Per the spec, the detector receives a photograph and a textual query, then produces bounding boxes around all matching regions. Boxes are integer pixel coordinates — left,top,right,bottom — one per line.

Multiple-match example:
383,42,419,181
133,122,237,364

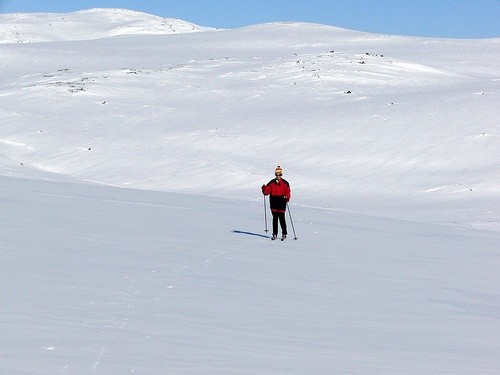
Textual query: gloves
285,197,289,203
262,185,266,191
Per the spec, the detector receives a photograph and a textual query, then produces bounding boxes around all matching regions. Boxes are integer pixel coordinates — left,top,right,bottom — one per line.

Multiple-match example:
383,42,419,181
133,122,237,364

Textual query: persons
261,165,291,241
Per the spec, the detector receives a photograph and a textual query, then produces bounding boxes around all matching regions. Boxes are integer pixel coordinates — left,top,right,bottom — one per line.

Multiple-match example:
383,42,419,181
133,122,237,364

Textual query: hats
275,166,283,173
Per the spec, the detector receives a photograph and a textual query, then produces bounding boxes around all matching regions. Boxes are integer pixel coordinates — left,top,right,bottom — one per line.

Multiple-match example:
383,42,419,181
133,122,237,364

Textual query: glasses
276,174,282,176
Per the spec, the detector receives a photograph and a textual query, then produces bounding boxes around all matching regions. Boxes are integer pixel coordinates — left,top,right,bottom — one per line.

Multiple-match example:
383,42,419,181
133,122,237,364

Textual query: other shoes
272,234,278,240
280,234,286,241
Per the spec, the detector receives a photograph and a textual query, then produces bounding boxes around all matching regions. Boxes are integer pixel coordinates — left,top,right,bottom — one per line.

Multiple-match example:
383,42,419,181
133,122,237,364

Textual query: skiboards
271,236,276,240
281,235,287,241
